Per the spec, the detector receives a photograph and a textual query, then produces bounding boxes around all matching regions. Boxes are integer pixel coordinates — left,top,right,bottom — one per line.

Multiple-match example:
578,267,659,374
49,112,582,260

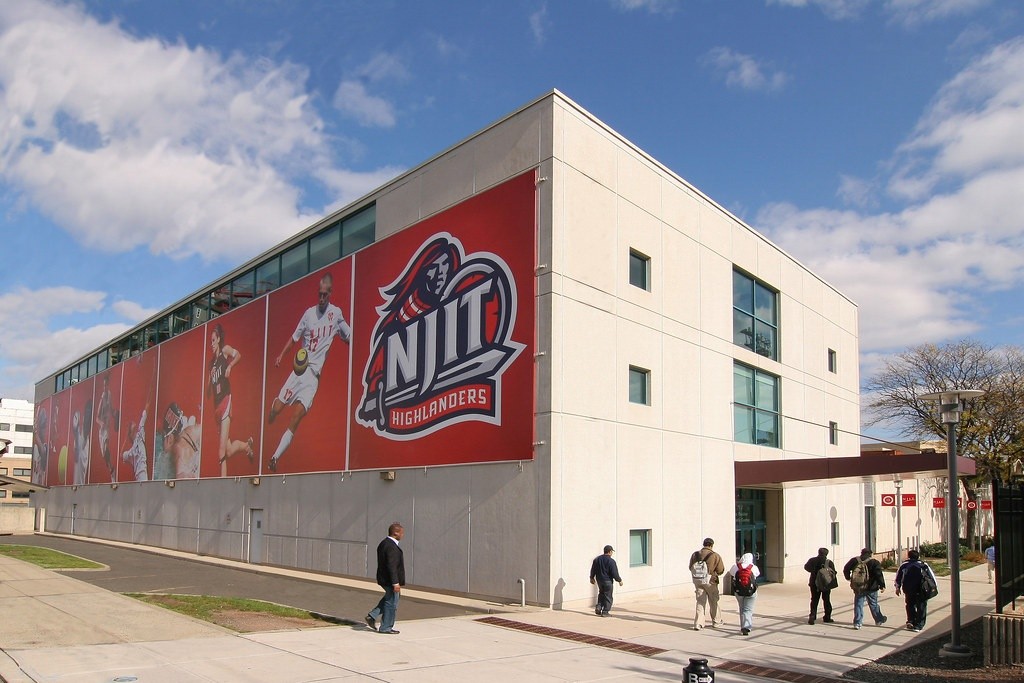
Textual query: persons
688,538,724,630
72,396,93,485
804,548,837,626
33,405,48,487
96,375,120,482
266,272,351,473
843,548,888,630
589,545,623,617
163,402,201,479
893,550,938,633
984,540,996,584
207,324,255,478
729,553,760,635
121,372,156,481
365,523,405,635
51,405,59,452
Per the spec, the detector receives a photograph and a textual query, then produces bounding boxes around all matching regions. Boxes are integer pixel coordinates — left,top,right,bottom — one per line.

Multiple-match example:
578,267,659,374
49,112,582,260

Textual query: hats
604,545,615,552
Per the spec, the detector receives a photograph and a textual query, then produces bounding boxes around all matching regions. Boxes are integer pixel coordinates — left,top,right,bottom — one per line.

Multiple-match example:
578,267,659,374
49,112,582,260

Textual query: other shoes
694,624,706,631
365,615,377,631
854,623,863,629
808,619,814,625
823,617,834,623
875,616,887,627
906,622,922,632
595,608,612,617
741,627,751,636
379,628,400,634
713,621,725,628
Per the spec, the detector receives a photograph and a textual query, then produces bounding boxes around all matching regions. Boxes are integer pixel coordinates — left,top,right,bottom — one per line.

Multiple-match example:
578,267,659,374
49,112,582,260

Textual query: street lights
971,488,987,554
894,479,904,567
917,389,986,658
942,481,948,566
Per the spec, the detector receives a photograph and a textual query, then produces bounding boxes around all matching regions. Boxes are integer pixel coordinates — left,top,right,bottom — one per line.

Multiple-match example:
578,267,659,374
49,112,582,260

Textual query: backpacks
732,564,758,596
850,556,874,591
815,559,838,592
691,551,714,585
911,563,938,601
